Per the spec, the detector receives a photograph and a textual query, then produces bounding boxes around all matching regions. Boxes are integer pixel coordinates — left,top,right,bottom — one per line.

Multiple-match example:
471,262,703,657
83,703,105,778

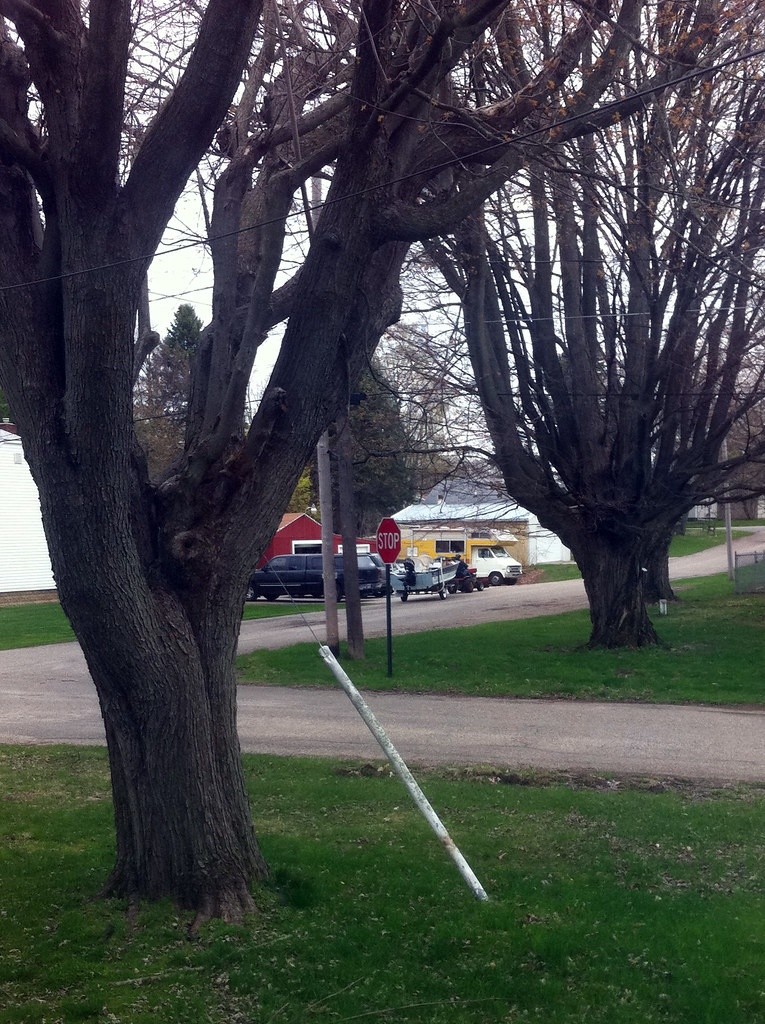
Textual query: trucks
398,527,522,586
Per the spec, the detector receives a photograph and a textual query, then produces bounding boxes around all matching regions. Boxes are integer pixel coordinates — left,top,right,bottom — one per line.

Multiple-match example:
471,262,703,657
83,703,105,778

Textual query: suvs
245,553,382,602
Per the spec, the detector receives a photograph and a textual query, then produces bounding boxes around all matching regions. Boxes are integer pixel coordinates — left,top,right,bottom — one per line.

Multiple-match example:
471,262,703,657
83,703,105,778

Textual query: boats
390,560,461,591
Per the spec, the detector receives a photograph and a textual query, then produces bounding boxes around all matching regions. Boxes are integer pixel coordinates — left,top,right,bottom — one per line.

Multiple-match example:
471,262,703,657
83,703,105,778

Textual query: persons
454,554,476,577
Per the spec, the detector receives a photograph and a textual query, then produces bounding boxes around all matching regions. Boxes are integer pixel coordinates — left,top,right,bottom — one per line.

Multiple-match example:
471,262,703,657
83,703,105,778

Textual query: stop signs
375,517,402,564
